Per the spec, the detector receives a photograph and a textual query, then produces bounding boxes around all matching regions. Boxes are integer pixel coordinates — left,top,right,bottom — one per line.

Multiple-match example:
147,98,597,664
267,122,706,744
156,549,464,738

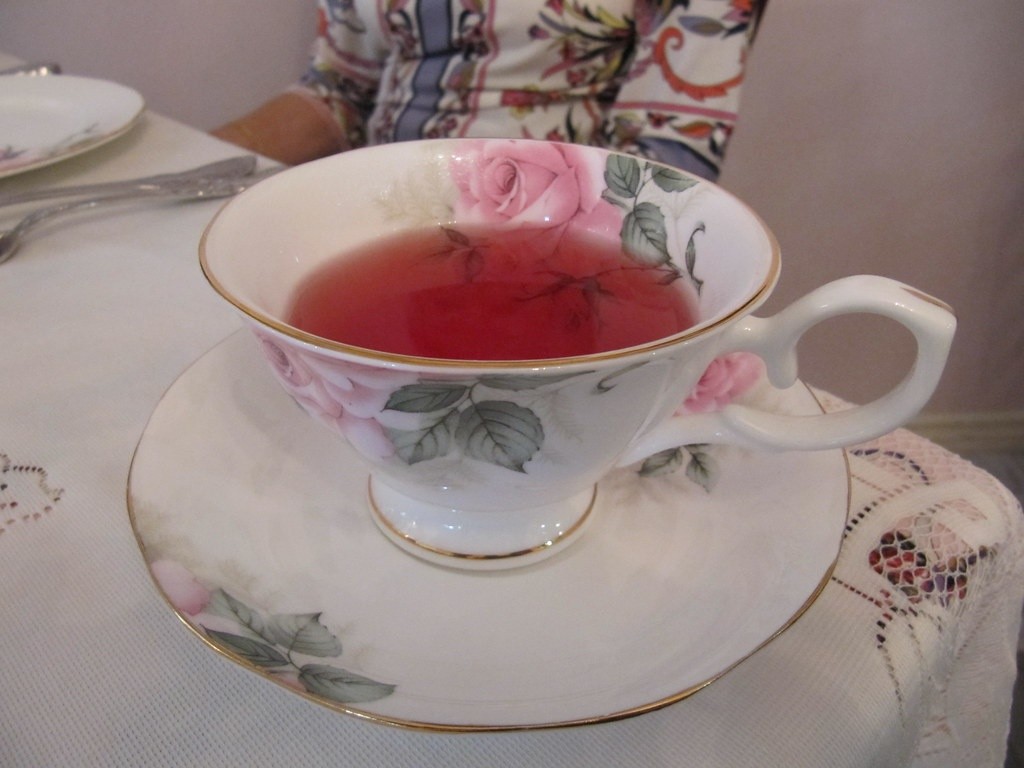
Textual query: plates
0,76,147,178
127,327,852,732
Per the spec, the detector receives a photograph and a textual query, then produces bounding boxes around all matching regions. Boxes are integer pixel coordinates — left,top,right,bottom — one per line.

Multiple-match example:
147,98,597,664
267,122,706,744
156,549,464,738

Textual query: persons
205,0,767,183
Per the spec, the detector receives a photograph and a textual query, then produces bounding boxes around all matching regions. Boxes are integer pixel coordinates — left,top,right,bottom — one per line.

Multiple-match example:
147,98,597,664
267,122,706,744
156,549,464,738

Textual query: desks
0,112,1024,768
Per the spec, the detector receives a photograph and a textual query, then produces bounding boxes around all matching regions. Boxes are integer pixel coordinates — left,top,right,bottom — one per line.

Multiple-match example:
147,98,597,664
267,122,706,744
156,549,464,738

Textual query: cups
198,137,958,570
